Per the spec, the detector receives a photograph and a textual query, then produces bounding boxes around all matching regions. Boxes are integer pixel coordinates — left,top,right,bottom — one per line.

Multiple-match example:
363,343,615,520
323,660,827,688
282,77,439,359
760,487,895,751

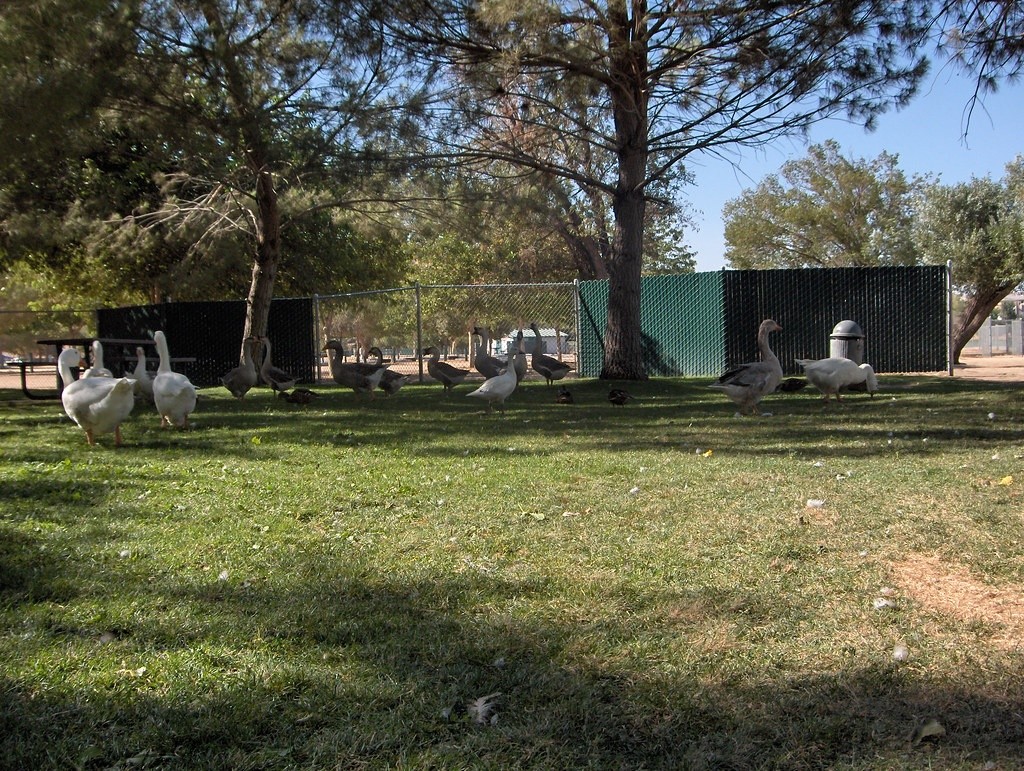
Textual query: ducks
56,317,877,448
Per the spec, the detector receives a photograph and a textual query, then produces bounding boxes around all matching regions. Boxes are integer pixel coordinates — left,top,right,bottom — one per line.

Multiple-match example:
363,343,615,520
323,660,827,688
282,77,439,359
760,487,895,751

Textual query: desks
37,337,157,401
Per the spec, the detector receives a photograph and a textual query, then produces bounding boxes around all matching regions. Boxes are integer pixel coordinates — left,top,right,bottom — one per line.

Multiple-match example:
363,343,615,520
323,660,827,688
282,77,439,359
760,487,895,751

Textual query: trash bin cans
830,319,865,366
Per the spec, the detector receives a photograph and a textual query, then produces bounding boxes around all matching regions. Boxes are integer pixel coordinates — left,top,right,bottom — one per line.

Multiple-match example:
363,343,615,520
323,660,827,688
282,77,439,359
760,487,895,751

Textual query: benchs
8,357,197,398
